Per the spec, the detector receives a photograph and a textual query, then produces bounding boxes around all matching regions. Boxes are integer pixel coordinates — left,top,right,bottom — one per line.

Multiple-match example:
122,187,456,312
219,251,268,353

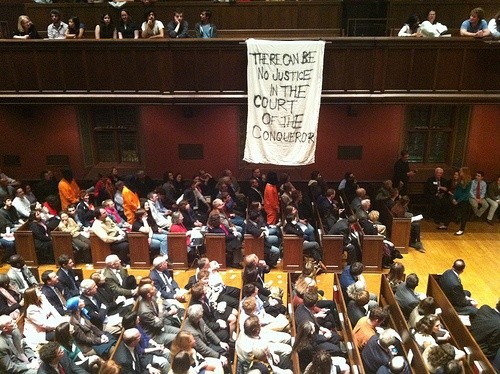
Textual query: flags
241,36,327,167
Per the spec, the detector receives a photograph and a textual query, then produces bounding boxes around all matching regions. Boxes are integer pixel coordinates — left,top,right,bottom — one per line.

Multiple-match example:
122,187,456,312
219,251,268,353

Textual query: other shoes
320,262,327,271
438,224,447,229
232,263,244,269
410,241,425,252
5,259,10,264
456,230,464,235
485,218,492,225
469,212,475,221
477,217,481,222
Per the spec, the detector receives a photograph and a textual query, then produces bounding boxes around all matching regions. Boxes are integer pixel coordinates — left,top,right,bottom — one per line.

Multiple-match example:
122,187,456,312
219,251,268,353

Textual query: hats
209,261,220,270
153,254,168,268
172,353,191,374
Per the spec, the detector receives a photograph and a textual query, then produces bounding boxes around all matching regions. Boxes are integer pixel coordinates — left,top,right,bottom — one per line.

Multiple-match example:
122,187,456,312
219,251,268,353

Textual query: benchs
15,192,500,373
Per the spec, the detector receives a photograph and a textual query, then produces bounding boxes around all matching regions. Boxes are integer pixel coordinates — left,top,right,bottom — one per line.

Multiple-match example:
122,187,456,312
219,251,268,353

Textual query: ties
21,268,33,288
92,296,100,309
162,272,177,294
58,363,66,374
54,286,67,307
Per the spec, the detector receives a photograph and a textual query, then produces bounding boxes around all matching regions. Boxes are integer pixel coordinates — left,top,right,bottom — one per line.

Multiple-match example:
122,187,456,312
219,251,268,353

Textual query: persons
451,166,472,236
425,168,454,229
420,8,448,38
485,176,500,226
469,171,489,221
398,14,424,38
447,171,463,222
460,7,491,38
488,11,500,37
0,165,500,374
11,0,219,41
391,149,418,196
